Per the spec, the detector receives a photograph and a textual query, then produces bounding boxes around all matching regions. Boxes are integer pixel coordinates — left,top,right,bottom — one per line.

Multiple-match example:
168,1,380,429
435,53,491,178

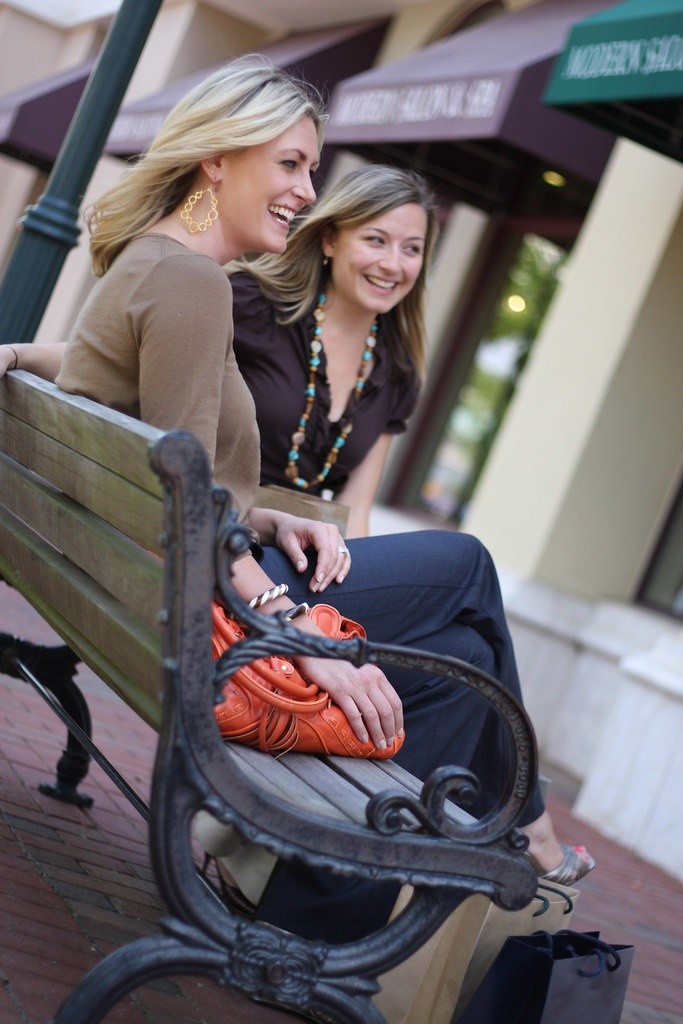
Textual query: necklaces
286,286,378,493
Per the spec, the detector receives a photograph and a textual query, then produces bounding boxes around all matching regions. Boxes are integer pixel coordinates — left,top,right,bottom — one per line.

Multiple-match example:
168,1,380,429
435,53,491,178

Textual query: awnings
0,56,96,170
309,0,610,210
540,0,683,159
104,18,386,157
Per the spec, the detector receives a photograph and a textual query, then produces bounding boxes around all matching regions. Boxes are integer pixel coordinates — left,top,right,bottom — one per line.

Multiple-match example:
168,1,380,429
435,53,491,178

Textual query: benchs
0,363,540,1024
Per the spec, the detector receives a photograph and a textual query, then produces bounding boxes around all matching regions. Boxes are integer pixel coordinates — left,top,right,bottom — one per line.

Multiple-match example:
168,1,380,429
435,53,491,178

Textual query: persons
0,59,598,1004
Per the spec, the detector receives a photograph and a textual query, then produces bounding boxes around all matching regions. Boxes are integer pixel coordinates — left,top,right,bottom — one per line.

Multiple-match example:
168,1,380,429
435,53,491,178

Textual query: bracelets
246,584,310,624
4,344,20,370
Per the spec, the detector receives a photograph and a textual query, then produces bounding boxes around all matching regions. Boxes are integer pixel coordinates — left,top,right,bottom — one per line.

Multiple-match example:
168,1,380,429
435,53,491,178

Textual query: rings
339,549,348,555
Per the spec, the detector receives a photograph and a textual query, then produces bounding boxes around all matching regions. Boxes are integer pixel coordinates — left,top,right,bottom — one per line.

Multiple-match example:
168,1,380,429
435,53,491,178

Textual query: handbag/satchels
210,598,406,760
456,928,636,1024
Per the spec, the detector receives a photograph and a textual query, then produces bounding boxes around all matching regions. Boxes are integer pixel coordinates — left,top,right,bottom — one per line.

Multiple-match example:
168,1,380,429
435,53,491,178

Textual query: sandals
537,845,597,888
